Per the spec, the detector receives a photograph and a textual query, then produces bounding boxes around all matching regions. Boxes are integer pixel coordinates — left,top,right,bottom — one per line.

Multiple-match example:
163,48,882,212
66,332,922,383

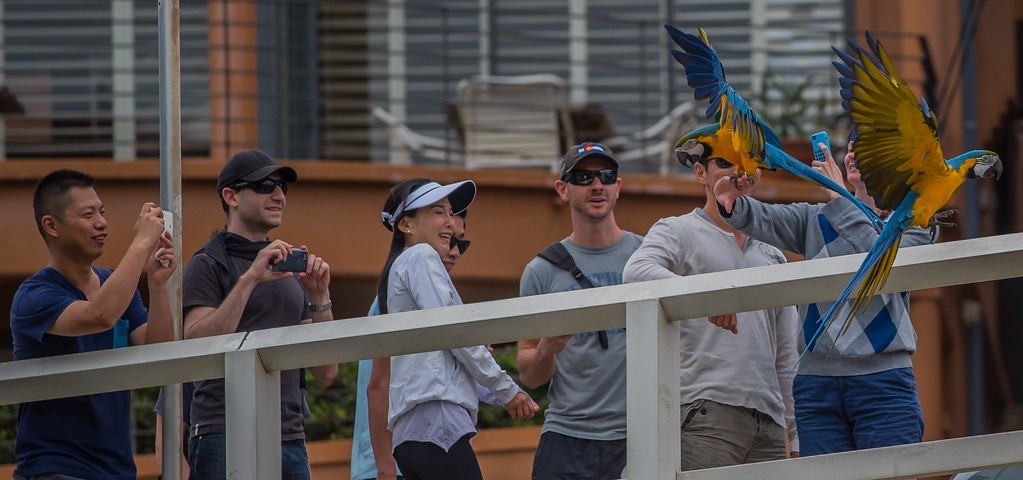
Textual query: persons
182,149,340,480
510,141,648,480
351,205,471,480
9,167,177,480
622,138,803,473
710,123,941,459
382,177,541,480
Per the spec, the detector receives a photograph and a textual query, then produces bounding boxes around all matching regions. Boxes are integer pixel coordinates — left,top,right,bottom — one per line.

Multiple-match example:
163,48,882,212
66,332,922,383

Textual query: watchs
308,298,332,314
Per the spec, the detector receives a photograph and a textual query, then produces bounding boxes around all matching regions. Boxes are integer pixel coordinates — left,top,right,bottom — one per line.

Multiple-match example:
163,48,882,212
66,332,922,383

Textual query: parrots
786,31,1003,379
664,24,886,236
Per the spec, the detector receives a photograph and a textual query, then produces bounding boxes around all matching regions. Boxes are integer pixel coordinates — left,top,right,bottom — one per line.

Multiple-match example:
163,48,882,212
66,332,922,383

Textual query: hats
381,180,476,231
560,141,620,182
215,149,296,195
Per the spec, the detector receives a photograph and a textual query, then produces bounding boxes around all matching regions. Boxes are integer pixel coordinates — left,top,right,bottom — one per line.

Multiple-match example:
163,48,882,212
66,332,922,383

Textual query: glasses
711,158,733,170
230,179,288,195
449,235,471,256
562,168,618,186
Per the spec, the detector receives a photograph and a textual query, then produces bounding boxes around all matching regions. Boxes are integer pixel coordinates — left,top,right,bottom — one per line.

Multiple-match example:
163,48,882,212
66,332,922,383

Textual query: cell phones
277,248,308,272
149,207,173,239
811,131,831,162
